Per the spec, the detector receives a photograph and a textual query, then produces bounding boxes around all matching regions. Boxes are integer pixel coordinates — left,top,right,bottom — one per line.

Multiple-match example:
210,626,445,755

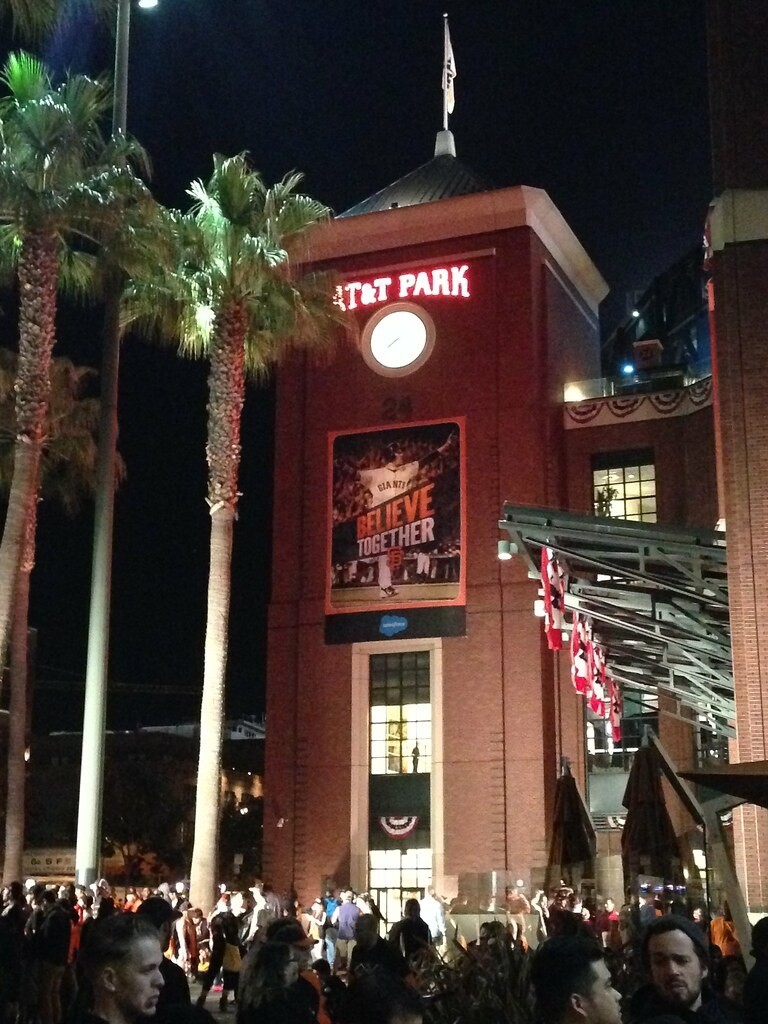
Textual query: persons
333,430,460,598
1,879,768,1024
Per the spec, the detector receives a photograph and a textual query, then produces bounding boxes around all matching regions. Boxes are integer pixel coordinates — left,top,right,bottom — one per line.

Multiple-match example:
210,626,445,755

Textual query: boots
220,998,235,1013
197,997,206,1008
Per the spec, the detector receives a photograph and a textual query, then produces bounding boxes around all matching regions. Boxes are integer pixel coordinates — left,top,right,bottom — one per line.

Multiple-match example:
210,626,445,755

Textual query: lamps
498,540,518,560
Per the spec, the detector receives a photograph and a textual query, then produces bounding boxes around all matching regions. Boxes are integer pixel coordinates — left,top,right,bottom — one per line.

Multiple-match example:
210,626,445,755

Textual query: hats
266,917,319,947
137,897,182,928
312,959,330,976
641,914,711,963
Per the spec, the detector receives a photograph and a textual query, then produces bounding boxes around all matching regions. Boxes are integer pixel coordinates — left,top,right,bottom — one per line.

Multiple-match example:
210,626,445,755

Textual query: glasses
293,945,310,951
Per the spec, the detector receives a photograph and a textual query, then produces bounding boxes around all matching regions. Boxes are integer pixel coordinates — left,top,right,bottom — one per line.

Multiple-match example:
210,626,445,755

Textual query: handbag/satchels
223,943,241,972
